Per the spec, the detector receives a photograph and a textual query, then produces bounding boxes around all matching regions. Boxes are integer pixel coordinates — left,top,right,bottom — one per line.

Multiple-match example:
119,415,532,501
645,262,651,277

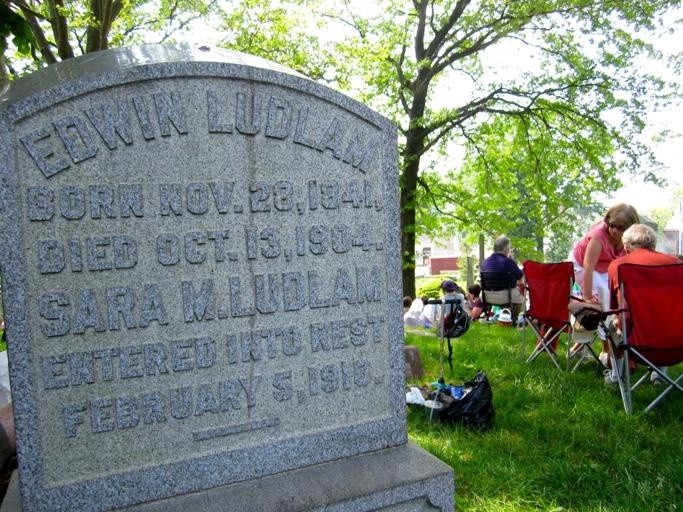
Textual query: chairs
481,261,683,414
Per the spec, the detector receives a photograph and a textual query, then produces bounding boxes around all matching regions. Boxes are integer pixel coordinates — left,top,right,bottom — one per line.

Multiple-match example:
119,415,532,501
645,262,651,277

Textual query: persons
601,222,682,387
400,278,484,322
566,203,640,368
479,235,526,326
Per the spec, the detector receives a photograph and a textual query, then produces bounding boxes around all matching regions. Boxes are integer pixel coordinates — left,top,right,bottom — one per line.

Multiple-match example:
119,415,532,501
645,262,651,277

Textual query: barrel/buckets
497,309,512,327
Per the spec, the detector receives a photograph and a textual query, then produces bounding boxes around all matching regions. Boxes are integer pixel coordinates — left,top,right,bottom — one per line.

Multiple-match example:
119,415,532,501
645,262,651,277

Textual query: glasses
607,222,625,230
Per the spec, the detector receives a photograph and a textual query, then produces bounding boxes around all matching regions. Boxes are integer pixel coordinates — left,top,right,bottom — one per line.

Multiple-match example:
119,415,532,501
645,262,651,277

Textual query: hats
441,280,458,292
570,314,594,343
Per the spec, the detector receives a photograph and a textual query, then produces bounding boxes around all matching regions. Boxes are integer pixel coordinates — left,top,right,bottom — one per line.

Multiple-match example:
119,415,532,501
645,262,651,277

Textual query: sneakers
598,352,607,368
602,369,621,386
650,370,668,386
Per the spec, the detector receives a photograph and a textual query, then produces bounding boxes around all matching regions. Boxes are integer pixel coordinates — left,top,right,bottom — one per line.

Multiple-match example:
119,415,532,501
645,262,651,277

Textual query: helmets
436,299,471,338
